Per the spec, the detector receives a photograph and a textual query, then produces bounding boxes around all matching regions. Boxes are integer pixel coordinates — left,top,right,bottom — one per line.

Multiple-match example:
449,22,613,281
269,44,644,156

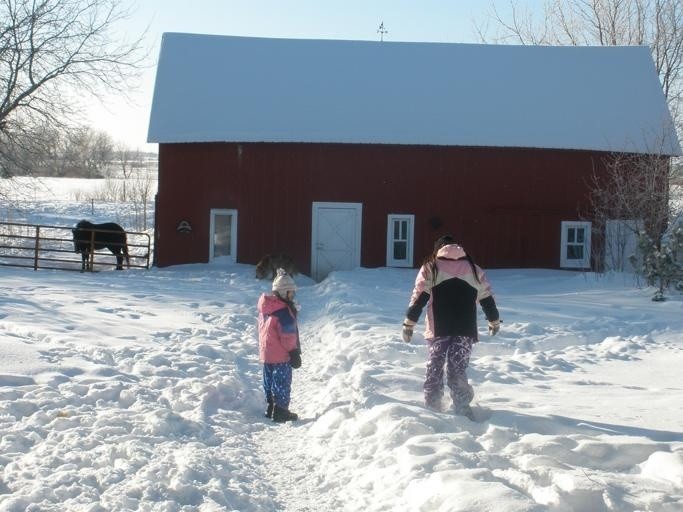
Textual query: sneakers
264,403,298,422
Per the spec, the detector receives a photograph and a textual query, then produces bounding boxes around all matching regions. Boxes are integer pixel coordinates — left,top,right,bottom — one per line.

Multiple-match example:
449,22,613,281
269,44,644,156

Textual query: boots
423,389,475,423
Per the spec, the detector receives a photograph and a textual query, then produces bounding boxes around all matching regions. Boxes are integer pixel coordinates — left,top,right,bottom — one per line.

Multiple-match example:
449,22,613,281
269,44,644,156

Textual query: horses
71,220,131,271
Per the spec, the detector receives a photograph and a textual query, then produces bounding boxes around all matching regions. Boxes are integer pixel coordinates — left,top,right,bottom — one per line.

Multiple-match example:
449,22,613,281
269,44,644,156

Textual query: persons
397,233,502,415
254,270,302,423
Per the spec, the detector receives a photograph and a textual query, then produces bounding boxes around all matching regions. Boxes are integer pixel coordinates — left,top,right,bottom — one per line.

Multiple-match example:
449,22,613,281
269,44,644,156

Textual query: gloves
289,348,302,369
402,317,418,343
487,318,501,337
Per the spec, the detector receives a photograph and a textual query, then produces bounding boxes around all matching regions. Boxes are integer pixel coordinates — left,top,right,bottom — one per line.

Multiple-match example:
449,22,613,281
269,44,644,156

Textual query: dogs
255,253,300,282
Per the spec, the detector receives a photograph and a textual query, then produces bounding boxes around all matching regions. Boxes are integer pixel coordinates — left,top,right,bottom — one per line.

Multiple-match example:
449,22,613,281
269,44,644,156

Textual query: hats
272,268,298,303
